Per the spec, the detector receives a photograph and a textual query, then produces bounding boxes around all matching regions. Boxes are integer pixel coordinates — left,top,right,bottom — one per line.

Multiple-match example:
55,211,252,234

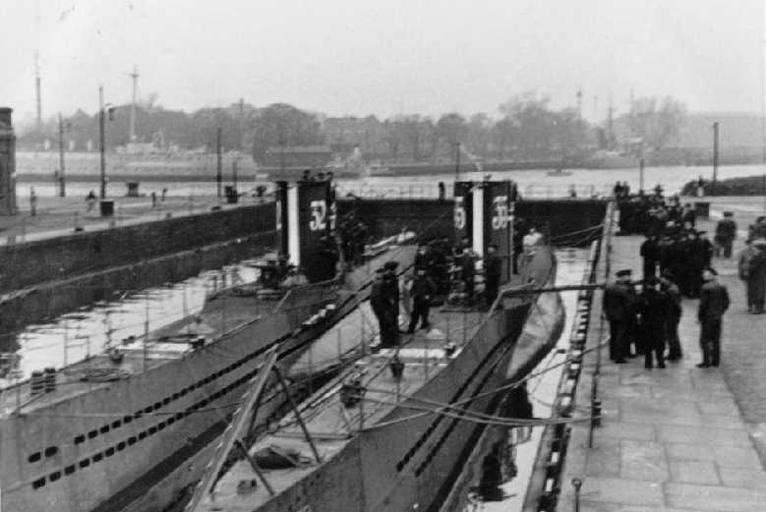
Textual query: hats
706,266,717,276
417,266,426,270
374,262,398,280
615,269,673,286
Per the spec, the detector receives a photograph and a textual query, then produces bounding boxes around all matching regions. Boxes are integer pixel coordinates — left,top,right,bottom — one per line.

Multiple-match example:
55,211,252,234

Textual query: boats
180,179,560,511
0,174,431,511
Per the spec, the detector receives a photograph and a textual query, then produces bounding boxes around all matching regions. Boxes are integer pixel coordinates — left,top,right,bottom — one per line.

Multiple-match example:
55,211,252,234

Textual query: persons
602,175,766,374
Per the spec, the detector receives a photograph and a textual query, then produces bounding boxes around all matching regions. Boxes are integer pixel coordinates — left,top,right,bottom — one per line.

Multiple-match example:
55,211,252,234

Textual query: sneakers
406,322,428,334
610,352,681,368
699,360,718,367
